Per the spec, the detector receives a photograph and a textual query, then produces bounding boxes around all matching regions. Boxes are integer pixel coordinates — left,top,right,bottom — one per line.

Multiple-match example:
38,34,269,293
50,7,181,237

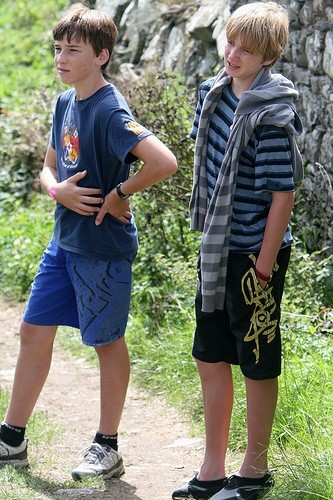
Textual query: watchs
255,268,272,282
116,182,133,200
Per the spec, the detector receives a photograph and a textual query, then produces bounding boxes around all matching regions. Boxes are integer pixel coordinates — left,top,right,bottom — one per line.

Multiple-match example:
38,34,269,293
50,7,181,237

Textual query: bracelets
50,184,56,201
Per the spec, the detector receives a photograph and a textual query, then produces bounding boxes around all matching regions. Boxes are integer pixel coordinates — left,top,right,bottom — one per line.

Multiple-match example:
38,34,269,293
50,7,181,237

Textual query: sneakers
72,441,125,480
0,438,29,466
172,477,229,500
209,473,275,500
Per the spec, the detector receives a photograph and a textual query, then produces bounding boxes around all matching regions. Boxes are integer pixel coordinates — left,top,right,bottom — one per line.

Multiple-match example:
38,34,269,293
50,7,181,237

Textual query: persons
0,4,178,482
171,2,304,500
